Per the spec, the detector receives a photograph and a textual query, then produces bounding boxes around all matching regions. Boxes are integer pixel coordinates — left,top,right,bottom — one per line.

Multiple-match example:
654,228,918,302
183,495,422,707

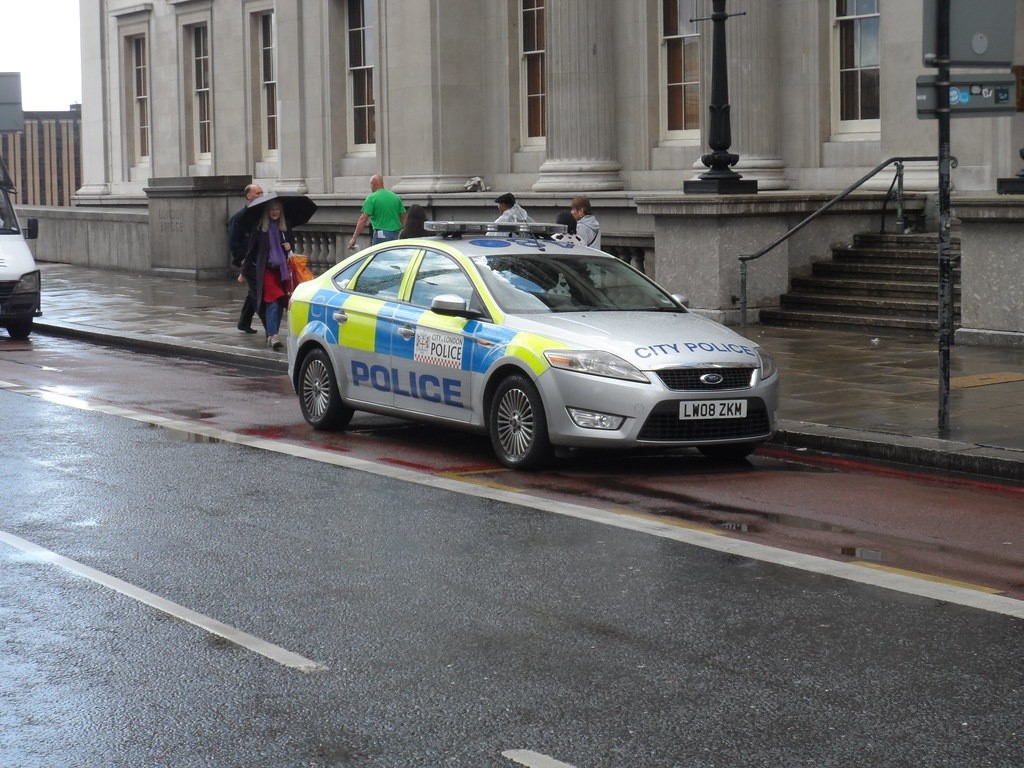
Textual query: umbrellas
244,191,319,243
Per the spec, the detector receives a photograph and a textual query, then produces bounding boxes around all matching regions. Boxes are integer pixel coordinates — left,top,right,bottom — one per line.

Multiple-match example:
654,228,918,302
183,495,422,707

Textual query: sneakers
268,334,283,348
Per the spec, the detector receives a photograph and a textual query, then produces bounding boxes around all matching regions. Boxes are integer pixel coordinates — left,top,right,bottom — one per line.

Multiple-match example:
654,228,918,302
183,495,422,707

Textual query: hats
495,193,515,206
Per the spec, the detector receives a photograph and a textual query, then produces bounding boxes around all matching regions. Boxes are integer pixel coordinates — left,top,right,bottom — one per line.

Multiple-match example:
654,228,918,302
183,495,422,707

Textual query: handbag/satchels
286,250,314,298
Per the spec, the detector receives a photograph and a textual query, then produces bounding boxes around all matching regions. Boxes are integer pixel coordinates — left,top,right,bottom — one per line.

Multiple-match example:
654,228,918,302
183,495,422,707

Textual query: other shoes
238,325,257,334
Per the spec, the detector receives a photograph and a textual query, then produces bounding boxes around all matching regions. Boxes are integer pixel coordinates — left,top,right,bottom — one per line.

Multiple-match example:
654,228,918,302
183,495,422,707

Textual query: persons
398,204,436,240
348,174,407,250
486,193,529,277
549,197,601,296
229,185,267,333
238,199,295,348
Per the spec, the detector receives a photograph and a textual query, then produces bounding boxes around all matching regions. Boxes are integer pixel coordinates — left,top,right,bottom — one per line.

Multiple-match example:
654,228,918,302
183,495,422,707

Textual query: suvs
0,156,41,342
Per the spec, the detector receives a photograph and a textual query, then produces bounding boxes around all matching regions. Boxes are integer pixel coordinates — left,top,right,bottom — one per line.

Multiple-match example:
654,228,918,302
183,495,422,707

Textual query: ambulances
287,221,779,470
